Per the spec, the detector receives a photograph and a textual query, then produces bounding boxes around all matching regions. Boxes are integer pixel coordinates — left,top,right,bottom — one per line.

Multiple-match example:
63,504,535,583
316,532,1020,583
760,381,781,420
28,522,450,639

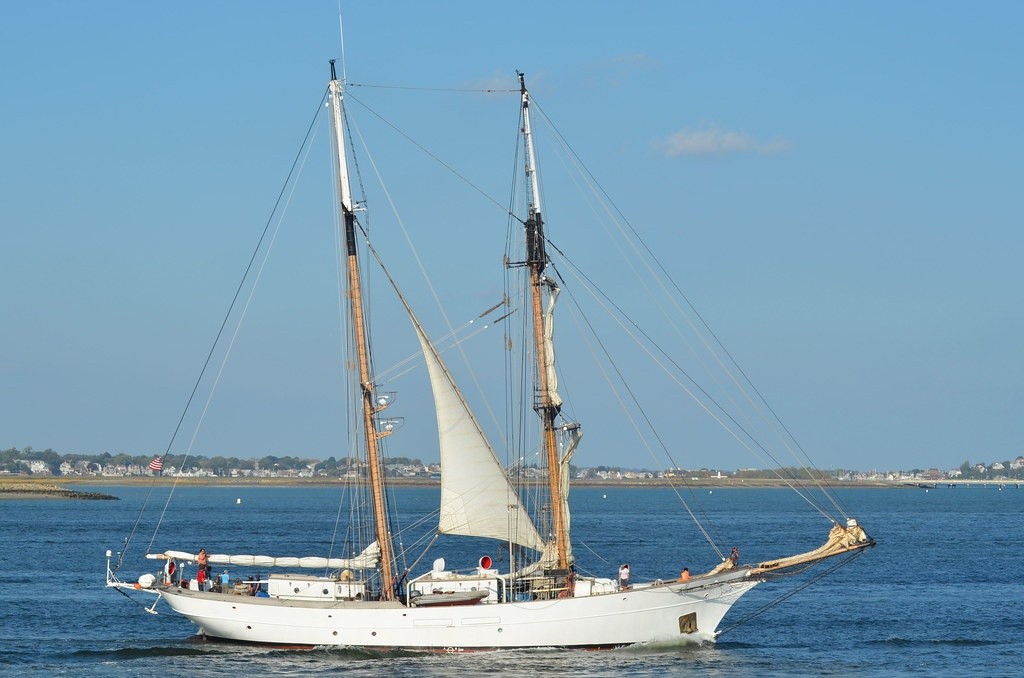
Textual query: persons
681,567,693,581
197,548,207,591
248,574,261,596
221,569,229,583
618,564,630,590
731,546,739,562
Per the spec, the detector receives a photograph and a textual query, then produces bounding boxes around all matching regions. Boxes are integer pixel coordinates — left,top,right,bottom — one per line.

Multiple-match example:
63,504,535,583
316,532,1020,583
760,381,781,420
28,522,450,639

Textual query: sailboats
101,54,877,648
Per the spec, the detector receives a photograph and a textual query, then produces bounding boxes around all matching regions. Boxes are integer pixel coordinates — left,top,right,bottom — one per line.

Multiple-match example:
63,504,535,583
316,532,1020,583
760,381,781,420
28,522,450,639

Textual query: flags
149,456,165,472
